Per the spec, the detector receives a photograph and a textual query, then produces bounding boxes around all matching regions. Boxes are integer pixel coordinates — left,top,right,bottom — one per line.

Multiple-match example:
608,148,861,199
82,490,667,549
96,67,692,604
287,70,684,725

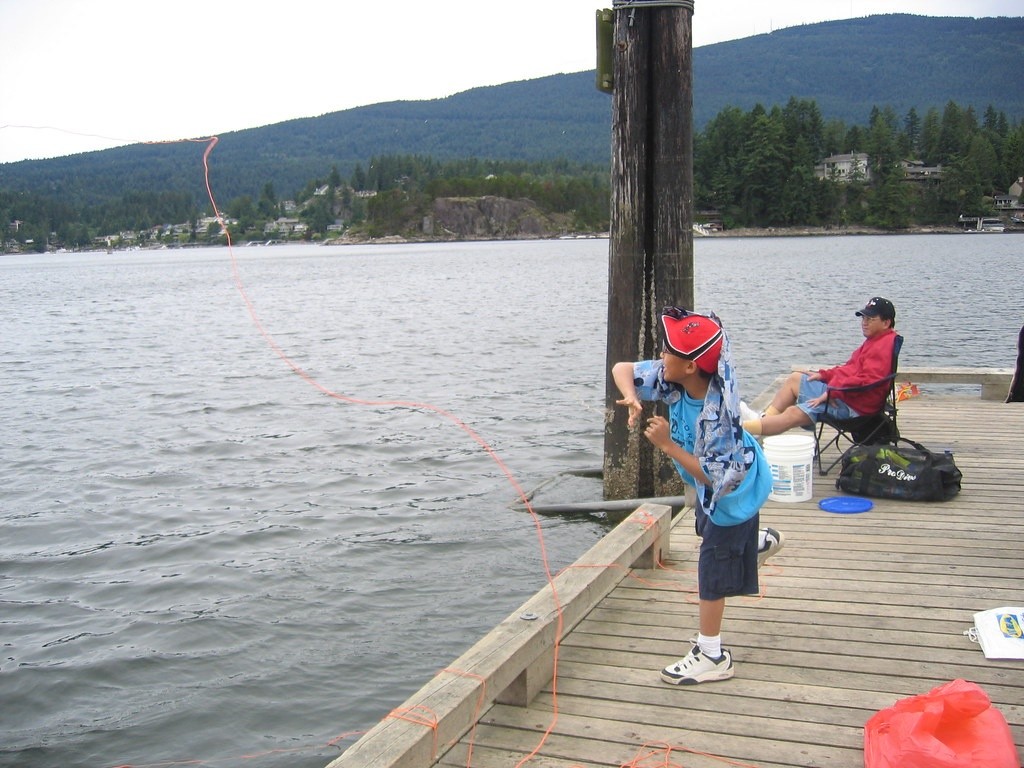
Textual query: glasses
662,339,672,354
862,315,880,321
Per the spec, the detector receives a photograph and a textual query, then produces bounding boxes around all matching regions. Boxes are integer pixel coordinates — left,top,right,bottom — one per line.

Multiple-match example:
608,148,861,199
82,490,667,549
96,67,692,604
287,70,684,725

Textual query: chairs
811,334,904,475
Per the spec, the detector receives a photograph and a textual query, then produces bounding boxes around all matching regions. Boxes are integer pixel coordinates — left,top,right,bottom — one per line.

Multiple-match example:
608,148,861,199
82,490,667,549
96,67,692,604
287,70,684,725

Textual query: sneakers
660,638,735,684
740,401,765,439
758,528,786,569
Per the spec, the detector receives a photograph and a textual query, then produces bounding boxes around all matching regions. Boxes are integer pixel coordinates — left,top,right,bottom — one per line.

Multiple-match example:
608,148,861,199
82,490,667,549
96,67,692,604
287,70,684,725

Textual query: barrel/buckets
762,435,817,503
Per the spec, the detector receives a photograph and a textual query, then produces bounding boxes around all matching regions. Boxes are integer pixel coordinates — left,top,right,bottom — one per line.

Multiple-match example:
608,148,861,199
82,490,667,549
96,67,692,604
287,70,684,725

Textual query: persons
611,313,785,686
739,296,901,436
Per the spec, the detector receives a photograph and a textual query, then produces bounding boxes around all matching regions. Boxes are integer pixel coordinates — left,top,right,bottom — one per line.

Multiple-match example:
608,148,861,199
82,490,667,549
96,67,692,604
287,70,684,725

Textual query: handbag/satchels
834,436,963,500
967,606,1024,660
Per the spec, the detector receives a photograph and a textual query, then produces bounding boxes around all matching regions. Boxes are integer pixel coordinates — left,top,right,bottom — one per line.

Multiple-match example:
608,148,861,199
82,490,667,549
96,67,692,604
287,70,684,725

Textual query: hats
660,315,723,374
855,296,896,318
